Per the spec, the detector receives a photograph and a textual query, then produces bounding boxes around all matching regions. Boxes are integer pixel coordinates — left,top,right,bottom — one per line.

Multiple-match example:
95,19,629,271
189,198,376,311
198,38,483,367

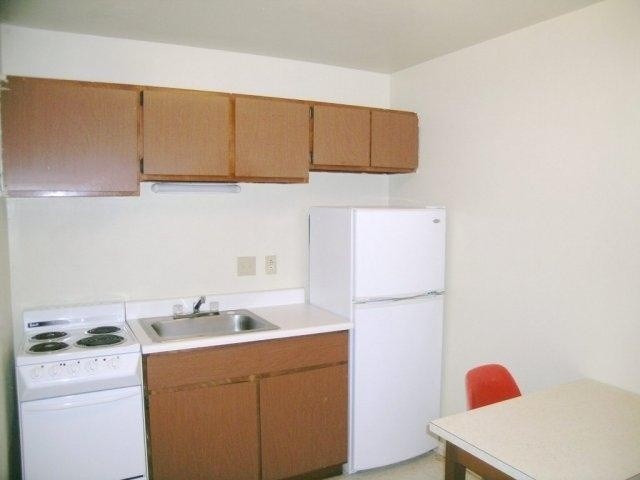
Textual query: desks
430,377,639,480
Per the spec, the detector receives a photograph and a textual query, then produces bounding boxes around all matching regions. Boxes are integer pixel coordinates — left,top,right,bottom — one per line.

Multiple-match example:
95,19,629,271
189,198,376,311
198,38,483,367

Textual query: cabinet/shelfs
145,333,349,479
139,86,311,184
0,77,141,200
310,101,419,174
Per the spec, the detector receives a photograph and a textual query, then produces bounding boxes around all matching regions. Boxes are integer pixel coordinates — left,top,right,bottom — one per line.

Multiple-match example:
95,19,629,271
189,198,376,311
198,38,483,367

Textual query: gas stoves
16,323,140,382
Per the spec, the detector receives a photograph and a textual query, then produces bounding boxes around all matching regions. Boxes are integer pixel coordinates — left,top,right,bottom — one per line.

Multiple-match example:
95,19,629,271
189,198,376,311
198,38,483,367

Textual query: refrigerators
308,204,446,476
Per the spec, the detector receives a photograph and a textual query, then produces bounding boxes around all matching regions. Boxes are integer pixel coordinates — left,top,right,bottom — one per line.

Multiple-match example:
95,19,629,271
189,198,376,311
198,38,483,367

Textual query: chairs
465,364,520,411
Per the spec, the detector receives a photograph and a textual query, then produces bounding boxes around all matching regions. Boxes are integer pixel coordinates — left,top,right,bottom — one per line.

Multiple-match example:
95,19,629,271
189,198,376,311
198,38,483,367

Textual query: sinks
138,310,281,345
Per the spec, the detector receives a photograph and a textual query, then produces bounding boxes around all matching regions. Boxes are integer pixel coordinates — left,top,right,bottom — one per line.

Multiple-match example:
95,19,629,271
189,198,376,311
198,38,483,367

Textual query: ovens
20,375,148,480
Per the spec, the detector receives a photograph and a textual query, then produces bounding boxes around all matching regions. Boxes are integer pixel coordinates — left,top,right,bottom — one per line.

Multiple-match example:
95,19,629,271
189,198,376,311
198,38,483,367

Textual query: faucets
193,295,206,314
